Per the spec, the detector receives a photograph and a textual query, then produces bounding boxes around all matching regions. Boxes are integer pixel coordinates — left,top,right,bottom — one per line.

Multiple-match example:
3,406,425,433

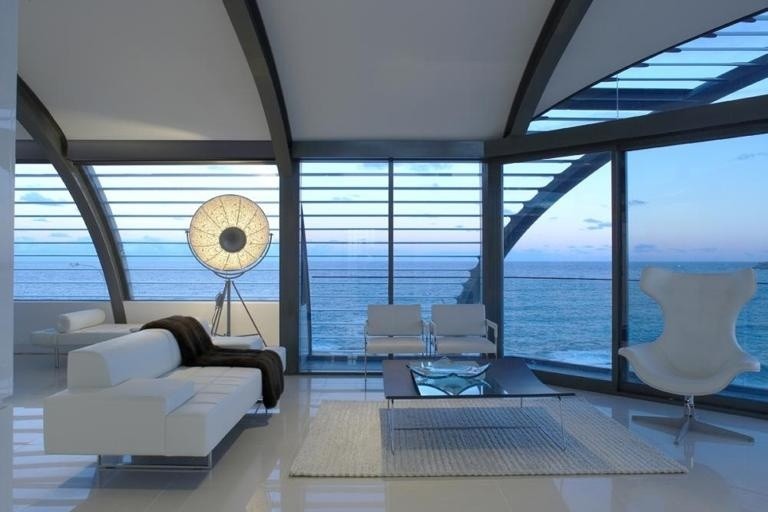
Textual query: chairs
428,303,498,360
365,304,430,399
616,268,760,445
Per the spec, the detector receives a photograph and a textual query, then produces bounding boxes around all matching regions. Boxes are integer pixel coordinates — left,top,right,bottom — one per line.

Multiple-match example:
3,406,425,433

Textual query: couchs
43,313,286,489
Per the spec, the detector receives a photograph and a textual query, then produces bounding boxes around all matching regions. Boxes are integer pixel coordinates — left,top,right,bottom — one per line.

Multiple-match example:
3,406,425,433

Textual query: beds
32,322,261,369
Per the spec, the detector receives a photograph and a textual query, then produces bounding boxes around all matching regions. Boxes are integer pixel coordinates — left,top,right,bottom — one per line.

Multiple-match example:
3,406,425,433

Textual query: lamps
186,195,273,348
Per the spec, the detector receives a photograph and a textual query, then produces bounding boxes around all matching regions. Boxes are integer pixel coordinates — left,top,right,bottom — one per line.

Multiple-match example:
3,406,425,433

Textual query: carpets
289,395,690,477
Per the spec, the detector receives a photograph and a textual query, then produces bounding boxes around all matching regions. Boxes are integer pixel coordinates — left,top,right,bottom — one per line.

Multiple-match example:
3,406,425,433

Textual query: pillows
55,308,106,333
